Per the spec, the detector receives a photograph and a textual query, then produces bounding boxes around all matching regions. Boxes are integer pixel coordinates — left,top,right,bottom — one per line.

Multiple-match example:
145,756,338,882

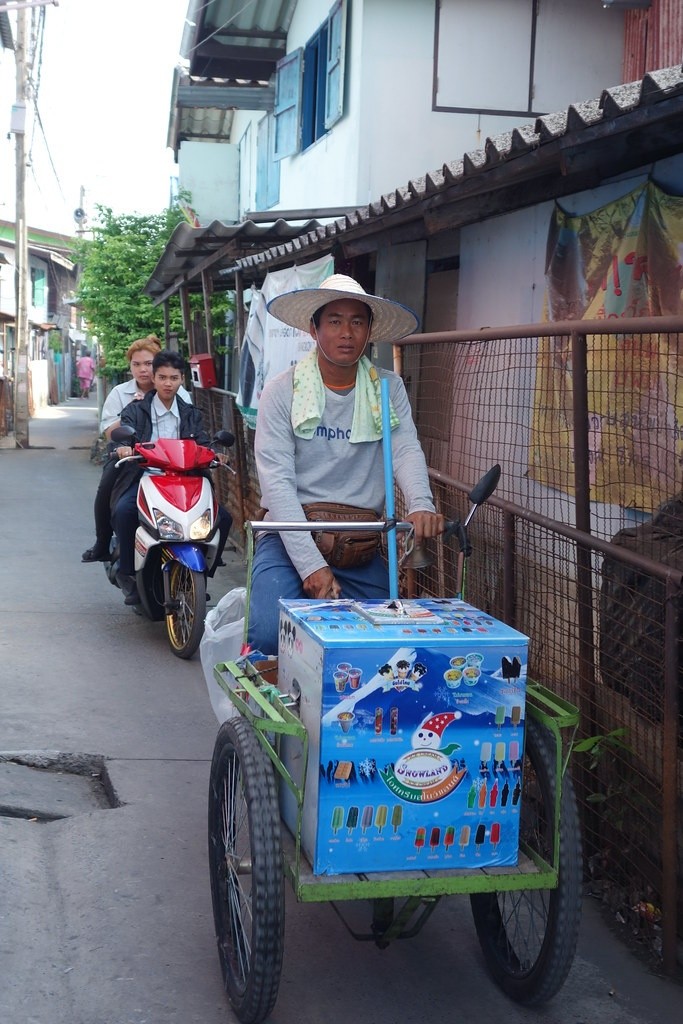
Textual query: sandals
81,546,110,563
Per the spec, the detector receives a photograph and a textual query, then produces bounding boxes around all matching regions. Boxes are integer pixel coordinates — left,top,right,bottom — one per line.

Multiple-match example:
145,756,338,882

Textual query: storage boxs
275,594,530,876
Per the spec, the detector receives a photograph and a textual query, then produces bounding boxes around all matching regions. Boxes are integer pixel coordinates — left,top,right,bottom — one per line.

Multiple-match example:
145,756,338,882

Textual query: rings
134,393,138,396
124,452,128,454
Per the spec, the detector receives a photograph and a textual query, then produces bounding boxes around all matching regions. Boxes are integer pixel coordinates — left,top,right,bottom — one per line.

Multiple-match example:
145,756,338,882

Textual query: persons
247,273,444,654
81,333,193,563
117,349,233,606
76,351,96,398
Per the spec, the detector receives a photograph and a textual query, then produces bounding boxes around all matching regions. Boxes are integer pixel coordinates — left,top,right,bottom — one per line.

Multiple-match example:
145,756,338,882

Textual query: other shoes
205,592,211,601
125,581,140,605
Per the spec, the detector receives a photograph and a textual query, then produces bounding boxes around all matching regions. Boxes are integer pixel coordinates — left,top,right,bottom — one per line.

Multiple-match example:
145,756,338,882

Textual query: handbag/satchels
302,502,383,570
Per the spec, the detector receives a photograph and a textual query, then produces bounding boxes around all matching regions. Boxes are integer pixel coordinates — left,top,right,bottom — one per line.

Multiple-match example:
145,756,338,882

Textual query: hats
266,273,419,345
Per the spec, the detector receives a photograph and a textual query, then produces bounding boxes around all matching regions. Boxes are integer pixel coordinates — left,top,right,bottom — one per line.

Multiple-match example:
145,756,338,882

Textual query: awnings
180,0,298,81
234,65,683,273
141,220,327,503
167,65,269,163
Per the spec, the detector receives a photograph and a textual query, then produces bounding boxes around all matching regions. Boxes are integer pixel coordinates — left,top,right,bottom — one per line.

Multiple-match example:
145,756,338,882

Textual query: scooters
106,423,241,660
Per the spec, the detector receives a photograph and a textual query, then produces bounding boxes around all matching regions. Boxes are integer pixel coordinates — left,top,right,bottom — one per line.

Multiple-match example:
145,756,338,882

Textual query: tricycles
206,463,587,1024
